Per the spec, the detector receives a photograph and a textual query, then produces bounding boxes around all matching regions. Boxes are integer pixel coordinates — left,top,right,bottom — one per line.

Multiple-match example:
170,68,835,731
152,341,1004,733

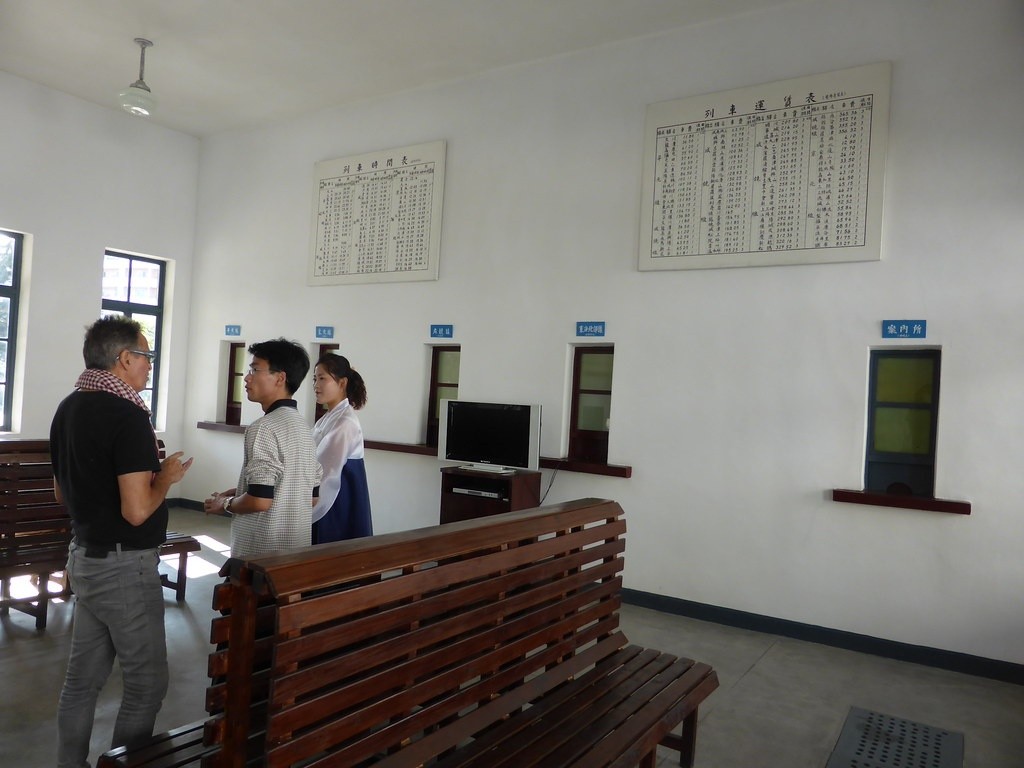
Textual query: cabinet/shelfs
436,466,541,592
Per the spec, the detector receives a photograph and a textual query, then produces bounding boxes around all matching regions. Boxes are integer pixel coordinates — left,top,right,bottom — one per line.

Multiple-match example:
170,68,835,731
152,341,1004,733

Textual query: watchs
223,496,236,515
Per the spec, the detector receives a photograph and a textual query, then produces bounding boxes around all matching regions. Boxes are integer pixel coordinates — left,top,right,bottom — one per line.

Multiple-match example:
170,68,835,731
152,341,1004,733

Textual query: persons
50,314,193,768
310,351,374,544
203,337,323,583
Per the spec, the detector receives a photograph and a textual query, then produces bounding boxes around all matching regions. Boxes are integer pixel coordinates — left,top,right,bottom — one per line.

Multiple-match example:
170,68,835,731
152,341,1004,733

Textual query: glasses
116,349,157,363
247,366,281,375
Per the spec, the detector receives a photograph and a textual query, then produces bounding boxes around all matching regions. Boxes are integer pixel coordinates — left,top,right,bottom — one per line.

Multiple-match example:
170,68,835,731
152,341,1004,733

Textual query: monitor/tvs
438,398,543,473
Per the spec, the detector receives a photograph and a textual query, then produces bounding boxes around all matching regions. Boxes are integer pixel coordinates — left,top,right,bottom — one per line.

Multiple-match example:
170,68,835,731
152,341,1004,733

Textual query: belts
73,539,153,551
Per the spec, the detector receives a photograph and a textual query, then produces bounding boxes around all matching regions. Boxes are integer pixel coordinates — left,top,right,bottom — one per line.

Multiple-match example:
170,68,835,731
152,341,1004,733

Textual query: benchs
94,499,720,768
0,439,202,630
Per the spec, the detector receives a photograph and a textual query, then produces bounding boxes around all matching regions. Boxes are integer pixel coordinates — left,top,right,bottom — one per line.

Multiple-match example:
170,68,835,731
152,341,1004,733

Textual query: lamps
118,39,155,116
